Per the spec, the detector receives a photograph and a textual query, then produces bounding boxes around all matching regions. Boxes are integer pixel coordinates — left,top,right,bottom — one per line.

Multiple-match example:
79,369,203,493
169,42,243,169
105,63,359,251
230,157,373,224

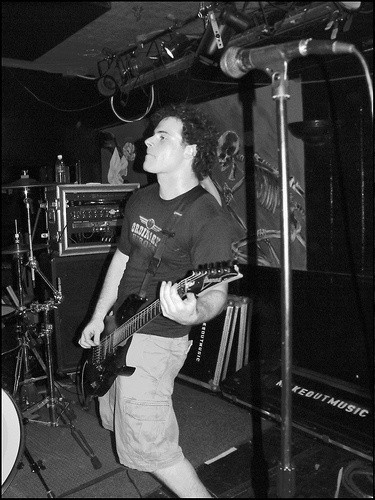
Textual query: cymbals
2,180,57,188
2,245,47,254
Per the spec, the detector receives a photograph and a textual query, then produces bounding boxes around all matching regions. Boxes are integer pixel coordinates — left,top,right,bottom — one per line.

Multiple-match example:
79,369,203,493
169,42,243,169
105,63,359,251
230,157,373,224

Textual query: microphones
220,37,354,79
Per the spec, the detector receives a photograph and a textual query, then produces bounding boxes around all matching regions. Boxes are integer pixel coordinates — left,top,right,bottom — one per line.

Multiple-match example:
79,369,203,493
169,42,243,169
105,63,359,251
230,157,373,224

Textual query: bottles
55,155,66,183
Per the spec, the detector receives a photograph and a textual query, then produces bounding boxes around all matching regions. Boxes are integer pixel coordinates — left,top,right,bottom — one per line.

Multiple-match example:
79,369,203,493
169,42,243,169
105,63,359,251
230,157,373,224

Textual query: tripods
11,170,102,470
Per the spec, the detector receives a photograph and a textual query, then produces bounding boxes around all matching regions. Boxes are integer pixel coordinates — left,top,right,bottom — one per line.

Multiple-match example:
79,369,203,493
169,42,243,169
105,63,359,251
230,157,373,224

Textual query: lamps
98,53,136,96
162,31,191,61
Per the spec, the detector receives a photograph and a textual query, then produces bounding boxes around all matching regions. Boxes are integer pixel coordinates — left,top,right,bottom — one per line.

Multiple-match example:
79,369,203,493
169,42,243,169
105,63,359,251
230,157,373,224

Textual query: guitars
78,264,242,399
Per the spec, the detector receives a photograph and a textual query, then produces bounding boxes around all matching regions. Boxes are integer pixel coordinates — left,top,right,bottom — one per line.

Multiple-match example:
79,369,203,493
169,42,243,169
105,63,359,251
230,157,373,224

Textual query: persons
76,112,238,498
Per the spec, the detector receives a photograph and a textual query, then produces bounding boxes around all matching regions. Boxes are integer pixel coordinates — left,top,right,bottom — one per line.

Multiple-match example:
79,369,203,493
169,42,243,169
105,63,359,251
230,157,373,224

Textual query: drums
0,306,21,355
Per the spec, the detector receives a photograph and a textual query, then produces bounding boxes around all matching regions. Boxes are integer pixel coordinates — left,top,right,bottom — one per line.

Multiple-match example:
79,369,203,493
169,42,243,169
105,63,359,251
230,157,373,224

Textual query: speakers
39,252,114,377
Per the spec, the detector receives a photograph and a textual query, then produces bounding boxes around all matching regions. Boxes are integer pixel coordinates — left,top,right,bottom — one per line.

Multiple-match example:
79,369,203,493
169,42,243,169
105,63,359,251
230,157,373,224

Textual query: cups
75,158,84,184
